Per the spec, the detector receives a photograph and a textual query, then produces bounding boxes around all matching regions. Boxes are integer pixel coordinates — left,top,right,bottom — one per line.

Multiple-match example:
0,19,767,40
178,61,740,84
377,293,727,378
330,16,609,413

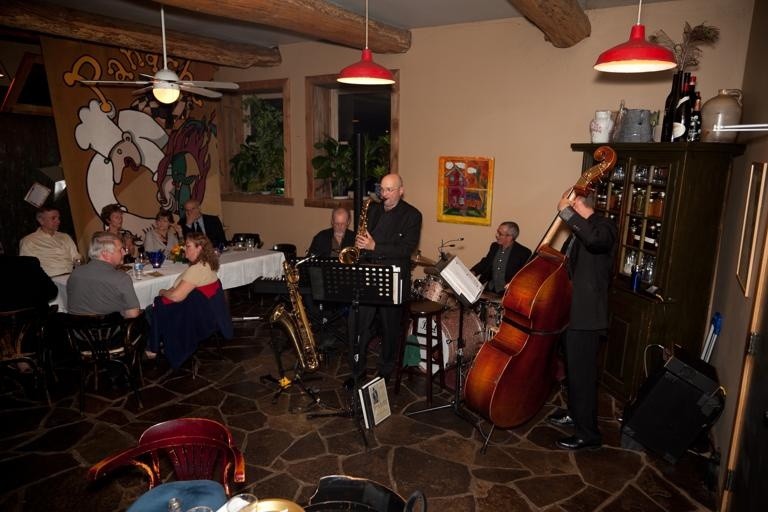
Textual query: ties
195,221,203,236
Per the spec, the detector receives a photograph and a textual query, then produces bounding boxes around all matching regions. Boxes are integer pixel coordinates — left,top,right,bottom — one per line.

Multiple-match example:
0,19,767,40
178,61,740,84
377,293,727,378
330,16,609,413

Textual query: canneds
596,195,606,208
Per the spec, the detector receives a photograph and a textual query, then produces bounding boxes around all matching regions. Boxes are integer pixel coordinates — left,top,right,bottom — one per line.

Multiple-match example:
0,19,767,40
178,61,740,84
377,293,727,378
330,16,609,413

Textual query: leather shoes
549,413,576,428
555,434,601,450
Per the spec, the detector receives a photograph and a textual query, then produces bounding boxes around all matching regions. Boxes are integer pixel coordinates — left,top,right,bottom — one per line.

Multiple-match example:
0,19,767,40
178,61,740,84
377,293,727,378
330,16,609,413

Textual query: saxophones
269,253,325,373
338,192,387,266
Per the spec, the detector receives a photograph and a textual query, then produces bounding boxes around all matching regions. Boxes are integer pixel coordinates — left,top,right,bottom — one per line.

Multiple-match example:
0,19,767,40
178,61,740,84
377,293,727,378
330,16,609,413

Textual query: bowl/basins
146,252,166,268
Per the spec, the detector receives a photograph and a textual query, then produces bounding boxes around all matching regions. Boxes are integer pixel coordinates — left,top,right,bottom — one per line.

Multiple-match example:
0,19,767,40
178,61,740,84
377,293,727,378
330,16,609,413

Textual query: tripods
307,301,370,447
272,318,328,404
405,302,489,438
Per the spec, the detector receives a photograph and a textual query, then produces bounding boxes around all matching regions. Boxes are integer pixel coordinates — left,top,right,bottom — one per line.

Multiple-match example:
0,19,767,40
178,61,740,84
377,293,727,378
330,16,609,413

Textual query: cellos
463,145,617,429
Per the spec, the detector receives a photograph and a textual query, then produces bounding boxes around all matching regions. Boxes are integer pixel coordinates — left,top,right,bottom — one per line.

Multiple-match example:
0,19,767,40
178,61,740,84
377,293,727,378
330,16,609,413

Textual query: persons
177,200,227,249
144,231,232,369
305,208,354,257
470,222,532,323
550,187,620,453
143,210,184,259
344,174,422,394
0,204,143,352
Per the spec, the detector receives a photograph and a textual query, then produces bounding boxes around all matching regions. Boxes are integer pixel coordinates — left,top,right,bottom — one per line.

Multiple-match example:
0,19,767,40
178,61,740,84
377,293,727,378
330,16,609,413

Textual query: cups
213,237,255,262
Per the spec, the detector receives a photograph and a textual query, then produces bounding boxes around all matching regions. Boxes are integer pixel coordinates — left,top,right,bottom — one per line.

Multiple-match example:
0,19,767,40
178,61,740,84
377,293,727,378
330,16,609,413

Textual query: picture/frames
436,154,495,227
733,159,768,299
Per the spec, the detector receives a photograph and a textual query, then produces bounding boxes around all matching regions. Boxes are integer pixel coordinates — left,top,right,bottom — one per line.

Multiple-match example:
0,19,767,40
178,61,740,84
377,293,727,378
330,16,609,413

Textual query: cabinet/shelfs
597,289,709,405
569,141,747,298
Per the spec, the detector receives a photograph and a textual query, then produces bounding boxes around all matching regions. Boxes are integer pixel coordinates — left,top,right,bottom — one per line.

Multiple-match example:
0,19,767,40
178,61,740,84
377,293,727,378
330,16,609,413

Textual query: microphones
308,253,320,260
438,238,464,260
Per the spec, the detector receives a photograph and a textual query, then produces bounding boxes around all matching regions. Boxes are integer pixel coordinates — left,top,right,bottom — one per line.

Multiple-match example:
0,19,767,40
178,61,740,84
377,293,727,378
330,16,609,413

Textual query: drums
408,306,486,376
413,275,451,306
470,291,502,333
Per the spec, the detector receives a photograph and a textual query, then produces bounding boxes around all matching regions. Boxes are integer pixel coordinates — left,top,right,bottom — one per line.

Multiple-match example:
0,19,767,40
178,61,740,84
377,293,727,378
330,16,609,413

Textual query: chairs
232,232,262,306
57,311,148,418
85,417,249,512
301,471,415,512
149,279,234,373
0,302,59,409
272,244,297,307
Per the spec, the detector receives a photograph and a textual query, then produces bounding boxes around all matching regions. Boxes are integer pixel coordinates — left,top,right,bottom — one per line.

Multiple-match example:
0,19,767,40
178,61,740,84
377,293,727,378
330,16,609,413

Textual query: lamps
592,1,679,79
332,1,397,89
151,84,180,104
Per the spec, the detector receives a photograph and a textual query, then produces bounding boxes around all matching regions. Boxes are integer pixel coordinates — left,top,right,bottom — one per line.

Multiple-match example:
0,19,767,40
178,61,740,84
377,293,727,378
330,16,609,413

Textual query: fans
78,8,239,103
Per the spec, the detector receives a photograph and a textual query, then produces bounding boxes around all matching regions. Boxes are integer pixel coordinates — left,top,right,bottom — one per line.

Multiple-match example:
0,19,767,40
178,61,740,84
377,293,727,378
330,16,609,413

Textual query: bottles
589,70,744,144
135,255,144,280
597,163,669,291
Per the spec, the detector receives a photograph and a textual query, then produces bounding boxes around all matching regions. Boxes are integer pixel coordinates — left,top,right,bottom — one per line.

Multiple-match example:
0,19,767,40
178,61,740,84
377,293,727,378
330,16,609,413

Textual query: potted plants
227,142,265,194
362,129,392,198
311,132,372,201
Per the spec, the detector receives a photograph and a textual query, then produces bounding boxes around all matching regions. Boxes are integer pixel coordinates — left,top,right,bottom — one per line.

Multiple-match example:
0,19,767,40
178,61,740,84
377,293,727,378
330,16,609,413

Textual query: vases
701,88,743,142
589,110,614,144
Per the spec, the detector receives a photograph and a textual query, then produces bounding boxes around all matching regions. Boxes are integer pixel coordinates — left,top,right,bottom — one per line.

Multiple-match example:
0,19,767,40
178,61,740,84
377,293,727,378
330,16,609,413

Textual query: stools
393,301,448,410
